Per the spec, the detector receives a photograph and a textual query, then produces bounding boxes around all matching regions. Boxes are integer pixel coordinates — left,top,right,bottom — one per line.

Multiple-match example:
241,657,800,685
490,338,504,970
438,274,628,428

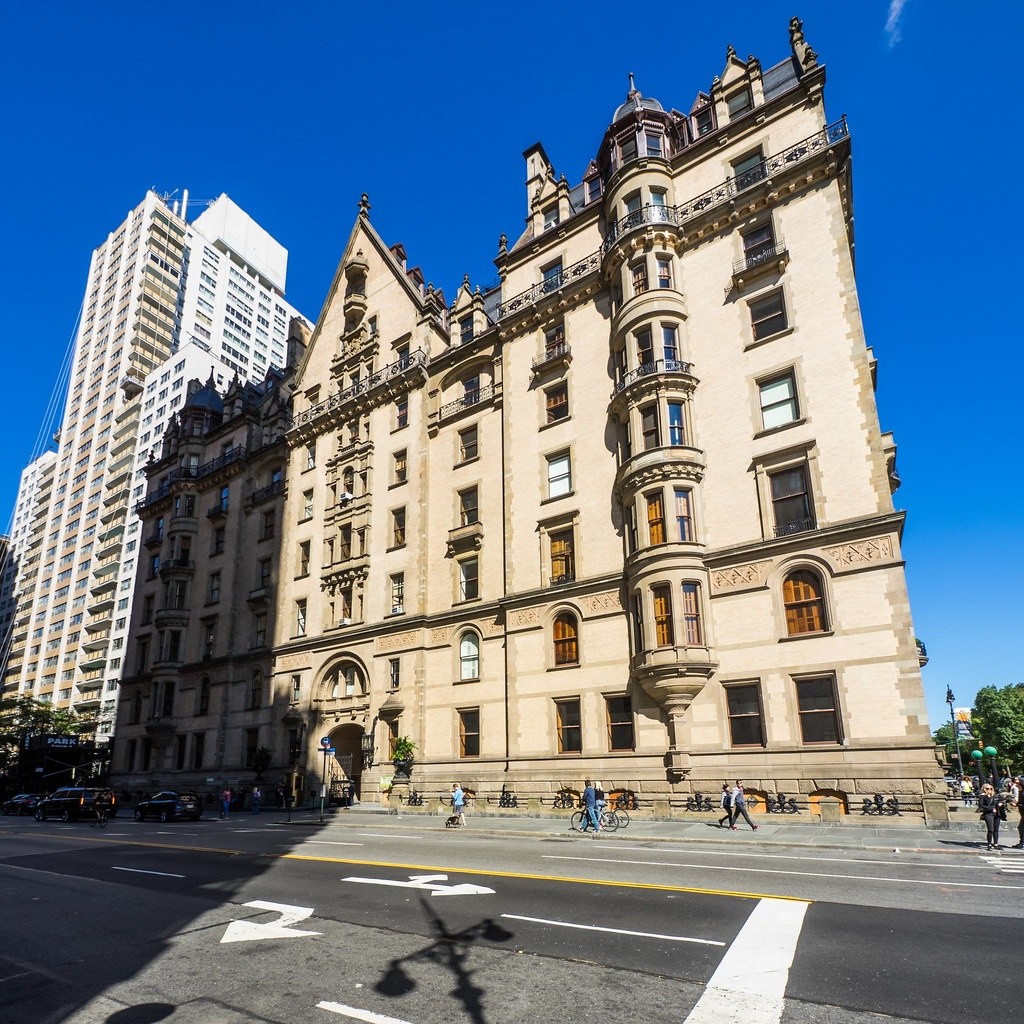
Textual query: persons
961,776,974,807
349,780,355,806
1012,780,1024,849
718,784,737,829
221,787,231,819
978,784,1004,851
728,779,759,831
594,781,606,821
450,784,466,826
576,780,602,833
253,787,261,815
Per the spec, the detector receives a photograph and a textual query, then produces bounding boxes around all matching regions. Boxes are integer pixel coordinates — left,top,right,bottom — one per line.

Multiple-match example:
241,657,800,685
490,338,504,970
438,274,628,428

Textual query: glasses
986,788,993,790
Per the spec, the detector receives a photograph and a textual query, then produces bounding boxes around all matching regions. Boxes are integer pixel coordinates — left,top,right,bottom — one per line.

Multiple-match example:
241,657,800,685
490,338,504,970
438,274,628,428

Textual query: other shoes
970,805,973,807
994,846,1003,850
1012,844,1024,850
719,820,723,826
97,817,102,821
752,826,758,830
988,846,993,850
578,828,584,833
966,804,969,807
729,828,734,831
731,826,737,831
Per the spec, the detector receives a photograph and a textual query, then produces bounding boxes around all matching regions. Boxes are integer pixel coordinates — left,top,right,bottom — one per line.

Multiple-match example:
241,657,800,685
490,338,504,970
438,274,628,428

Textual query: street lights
945,683,964,777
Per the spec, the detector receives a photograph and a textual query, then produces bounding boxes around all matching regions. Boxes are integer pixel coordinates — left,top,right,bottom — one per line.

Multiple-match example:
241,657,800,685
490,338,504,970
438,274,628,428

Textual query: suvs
33,786,119,824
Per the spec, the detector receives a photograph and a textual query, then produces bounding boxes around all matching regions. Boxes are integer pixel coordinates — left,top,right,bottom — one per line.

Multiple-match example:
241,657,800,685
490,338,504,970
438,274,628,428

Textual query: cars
135,790,203,823
1,794,48,817
944,774,1024,800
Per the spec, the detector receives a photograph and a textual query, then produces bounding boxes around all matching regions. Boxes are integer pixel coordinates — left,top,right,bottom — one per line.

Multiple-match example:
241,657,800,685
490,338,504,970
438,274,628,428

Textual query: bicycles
588,801,629,828
88,803,112,828
571,801,619,832
